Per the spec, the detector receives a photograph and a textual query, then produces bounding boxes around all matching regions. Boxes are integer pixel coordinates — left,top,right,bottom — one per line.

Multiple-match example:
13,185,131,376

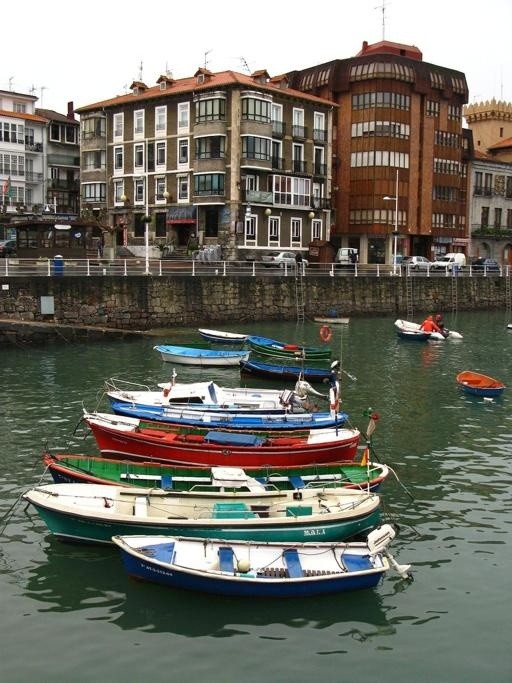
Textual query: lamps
307,212,315,224
121,195,129,202
263,208,272,224
163,191,173,201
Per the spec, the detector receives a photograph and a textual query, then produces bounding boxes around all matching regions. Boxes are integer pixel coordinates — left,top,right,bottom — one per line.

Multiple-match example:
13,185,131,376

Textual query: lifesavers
320,324,332,343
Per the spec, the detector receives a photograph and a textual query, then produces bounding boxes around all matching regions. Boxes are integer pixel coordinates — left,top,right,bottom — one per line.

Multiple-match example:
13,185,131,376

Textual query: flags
3,175,9,192
8,176,14,198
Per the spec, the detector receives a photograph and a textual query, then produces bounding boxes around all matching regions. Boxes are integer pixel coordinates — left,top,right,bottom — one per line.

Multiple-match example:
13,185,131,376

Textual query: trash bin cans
51,255,63,275
393,254,402,264
453,265,458,276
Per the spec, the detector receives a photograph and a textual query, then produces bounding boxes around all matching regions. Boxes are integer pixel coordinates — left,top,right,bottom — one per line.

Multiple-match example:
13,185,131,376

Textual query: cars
260,251,309,268
471,258,501,273
400,256,436,270
0,240,17,258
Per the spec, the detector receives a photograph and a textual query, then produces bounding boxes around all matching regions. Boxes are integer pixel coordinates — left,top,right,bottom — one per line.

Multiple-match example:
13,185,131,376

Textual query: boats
21,483,381,547
314,317,350,324
393,318,464,340
44,452,388,492
153,345,252,368
247,335,332,362
102,367,330,415
82,406,362,467
198,328,248,343
111,523,415,600
110,401,349,431
239,360,358,384
455,368,507,397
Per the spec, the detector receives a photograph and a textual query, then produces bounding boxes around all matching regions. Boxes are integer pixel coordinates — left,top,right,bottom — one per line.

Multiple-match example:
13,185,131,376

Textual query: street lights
44,197,58,213
383,169,398,274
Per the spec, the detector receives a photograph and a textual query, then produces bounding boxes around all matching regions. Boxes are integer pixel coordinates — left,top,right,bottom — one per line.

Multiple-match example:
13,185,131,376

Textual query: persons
418,315,443,333
348,249,359,268
295,249,302,274
435,314,443,332
187,233,198,256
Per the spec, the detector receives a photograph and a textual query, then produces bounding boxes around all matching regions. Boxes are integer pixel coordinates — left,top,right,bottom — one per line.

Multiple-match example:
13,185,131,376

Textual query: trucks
435,256,466,271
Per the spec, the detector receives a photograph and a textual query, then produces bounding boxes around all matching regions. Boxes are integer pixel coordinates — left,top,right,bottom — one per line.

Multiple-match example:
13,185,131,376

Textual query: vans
335,247,359,268
